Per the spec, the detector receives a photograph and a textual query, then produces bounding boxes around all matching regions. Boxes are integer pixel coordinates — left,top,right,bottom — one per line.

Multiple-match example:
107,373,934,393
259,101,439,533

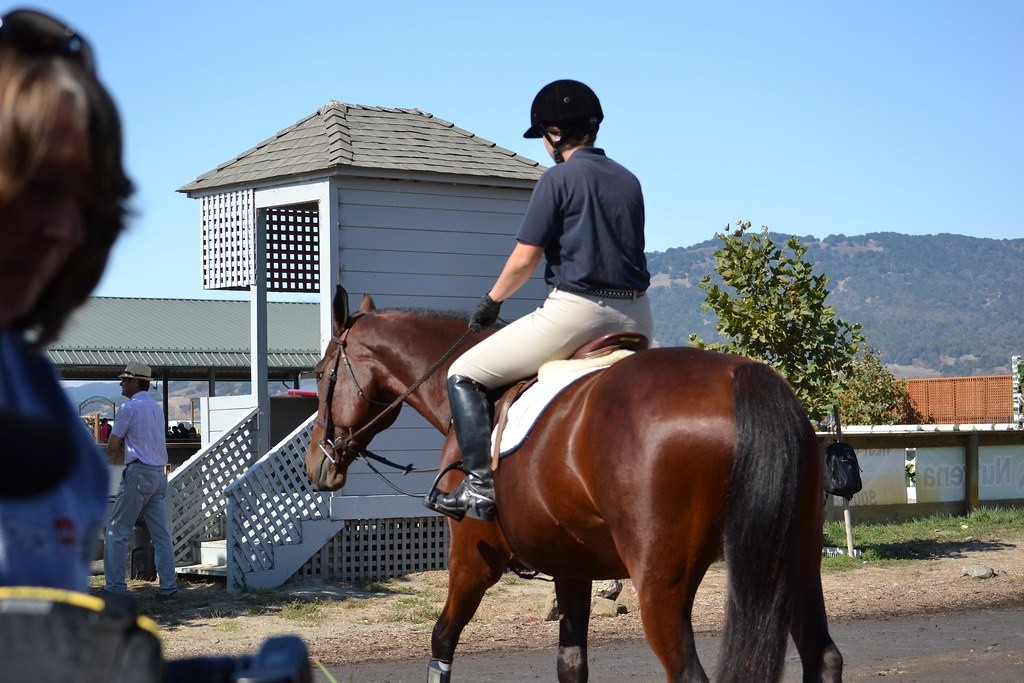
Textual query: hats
119,361,151,380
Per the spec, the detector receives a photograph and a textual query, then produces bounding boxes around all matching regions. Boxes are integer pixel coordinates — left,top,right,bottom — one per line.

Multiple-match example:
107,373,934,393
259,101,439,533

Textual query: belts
133,459,139,463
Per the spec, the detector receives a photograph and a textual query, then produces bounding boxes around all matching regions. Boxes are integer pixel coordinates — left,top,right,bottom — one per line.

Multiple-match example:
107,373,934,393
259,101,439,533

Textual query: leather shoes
92,588,114,597
155,589,179,601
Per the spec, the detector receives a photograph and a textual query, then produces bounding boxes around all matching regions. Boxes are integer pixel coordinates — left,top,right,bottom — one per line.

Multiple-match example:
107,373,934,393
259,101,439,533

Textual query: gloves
467,293,503,329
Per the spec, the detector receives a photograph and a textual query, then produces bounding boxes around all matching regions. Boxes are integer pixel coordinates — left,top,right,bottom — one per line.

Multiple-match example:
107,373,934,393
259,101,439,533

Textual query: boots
433,374,499,520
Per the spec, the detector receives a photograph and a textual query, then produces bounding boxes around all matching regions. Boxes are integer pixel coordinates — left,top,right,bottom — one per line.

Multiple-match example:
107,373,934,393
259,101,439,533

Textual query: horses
302,283,846,683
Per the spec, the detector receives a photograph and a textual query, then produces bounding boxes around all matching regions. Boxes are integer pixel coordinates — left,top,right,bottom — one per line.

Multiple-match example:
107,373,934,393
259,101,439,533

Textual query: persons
101,418,112,442
431,80,654,519
0,9,136,590
103,362,177,601
168,423,189,437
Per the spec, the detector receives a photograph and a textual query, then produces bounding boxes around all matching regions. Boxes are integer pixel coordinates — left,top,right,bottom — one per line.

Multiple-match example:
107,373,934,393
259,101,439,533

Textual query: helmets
524,78,604,141
